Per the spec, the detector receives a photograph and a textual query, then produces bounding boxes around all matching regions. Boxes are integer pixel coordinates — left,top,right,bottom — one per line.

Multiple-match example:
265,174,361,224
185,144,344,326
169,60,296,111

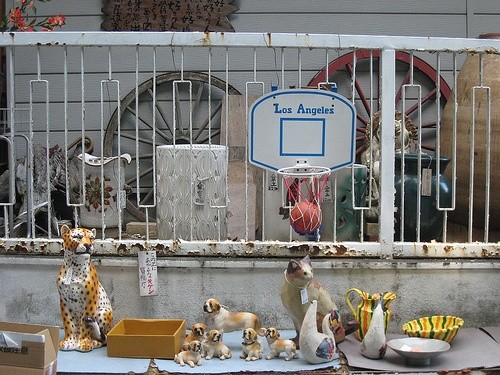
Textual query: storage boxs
107,317,186,360
0,358,57,375
0,322,60,368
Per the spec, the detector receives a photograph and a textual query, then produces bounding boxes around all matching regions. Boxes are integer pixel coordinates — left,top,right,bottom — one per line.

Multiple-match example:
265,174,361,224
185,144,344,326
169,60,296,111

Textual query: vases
394,152,452,242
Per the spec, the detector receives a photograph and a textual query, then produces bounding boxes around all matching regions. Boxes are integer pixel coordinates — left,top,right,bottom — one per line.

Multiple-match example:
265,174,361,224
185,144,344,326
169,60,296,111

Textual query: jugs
346,288,395,342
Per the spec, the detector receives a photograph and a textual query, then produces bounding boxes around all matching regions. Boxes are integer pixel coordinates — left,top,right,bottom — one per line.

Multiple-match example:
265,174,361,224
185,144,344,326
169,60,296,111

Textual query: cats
279,255,362,350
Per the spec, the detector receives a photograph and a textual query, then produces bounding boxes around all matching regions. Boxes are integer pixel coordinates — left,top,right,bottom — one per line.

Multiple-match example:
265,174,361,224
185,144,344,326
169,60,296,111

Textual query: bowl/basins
387,338,450,366
402,316,464,343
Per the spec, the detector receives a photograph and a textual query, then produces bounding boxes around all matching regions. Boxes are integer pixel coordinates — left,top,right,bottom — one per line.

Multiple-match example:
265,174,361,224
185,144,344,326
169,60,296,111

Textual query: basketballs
290,201,322,235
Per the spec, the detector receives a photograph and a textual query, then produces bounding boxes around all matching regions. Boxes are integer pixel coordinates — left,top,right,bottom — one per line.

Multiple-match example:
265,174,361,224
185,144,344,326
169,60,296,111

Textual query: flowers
0,0,67,32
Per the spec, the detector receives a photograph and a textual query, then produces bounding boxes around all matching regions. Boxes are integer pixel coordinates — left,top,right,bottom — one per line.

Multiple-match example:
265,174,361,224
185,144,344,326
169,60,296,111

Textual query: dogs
172,325,296,368
203,298,266,346
